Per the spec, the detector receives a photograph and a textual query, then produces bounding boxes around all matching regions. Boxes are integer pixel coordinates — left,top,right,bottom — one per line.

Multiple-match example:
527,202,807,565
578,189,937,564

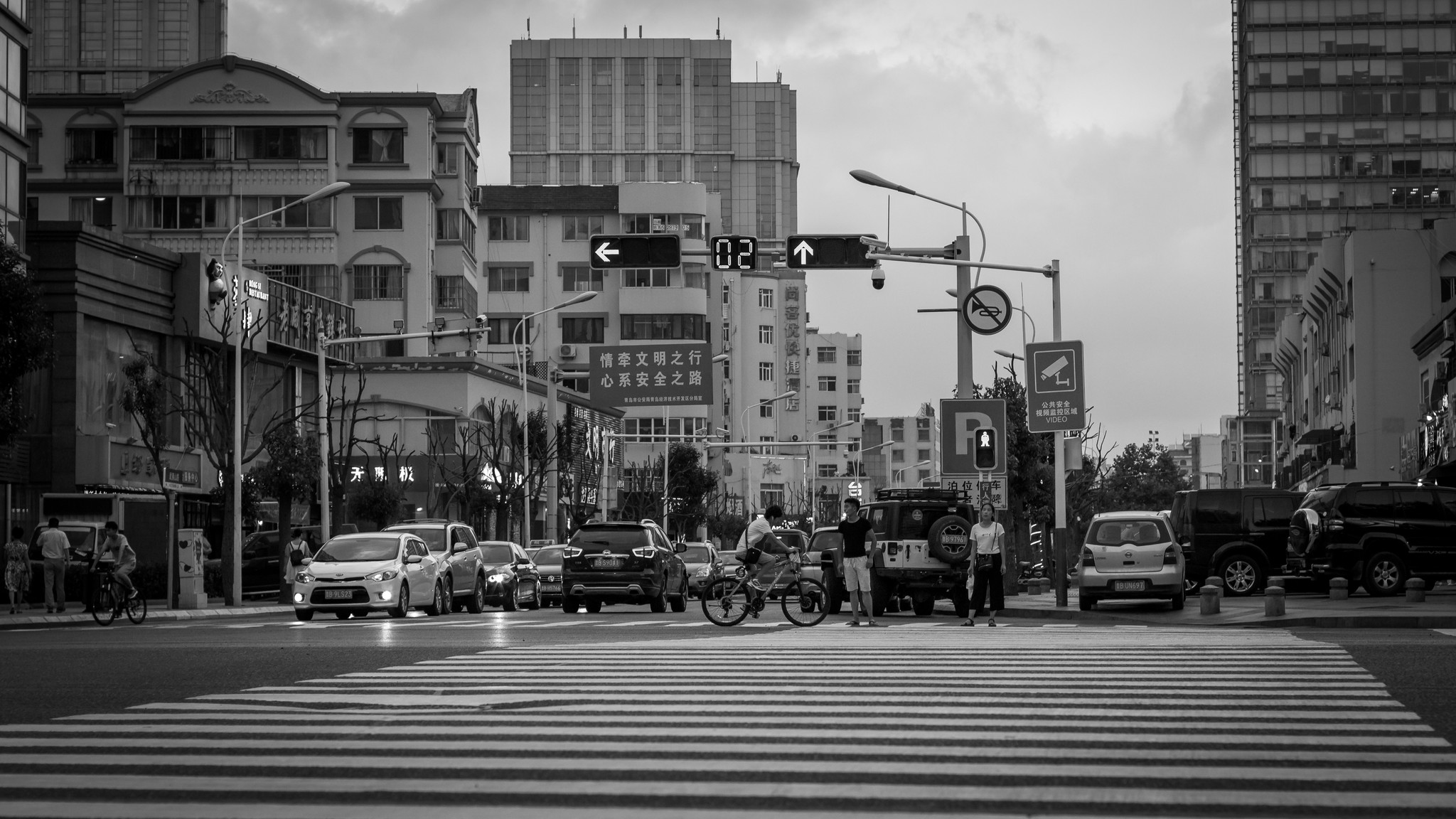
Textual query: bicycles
701,549,830,627
89,564,147,626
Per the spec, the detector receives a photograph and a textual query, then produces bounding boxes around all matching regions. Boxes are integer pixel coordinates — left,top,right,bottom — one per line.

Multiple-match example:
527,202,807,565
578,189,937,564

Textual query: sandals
988,619,996,627
961,618,975,626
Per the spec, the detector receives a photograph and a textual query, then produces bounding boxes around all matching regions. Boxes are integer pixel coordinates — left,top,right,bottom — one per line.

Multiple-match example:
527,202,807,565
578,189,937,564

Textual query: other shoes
47,609,53,613
128,589,138,599
111,614,123,618
10,607,14,614
17,610,23,613
56,608,66,613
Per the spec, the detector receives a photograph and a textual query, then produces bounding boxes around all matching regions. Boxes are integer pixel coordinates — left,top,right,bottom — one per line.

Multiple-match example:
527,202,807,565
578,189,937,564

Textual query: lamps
1390,465,1395,471
184,445,195,453
127,437,138,444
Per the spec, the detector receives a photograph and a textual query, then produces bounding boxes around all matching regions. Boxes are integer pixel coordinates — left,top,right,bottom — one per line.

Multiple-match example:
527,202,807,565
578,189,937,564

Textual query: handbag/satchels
966,574,974,600
746,547,760,564
978,555,993,570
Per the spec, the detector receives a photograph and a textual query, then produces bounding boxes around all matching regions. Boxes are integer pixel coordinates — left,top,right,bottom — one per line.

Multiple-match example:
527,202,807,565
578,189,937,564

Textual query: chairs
1137,525,1160,543
1098,525,1122,544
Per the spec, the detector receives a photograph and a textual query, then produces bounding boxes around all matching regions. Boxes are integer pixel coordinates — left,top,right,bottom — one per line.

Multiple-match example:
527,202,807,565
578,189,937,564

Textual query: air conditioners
806,347,810,356
1435,361,1445,380
559,343,576,356
1279,401,1287,412
1443,318,1455,338
1337,300,1345,313
790,434,802,441
1277,443,1288,459
1340,434,1350,448
519,344,531,354
499,446,510,462
806,312,810,323
828,423,837,429
470,186,482,205
1286,393,1291,402
724,342,729,351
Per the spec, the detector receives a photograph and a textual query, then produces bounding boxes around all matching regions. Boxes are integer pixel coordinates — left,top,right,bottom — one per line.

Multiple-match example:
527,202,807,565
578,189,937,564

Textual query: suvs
1176,480,1456,596
560,519,725,613
201,523,360,597
820,489,978,619
379,519,487,615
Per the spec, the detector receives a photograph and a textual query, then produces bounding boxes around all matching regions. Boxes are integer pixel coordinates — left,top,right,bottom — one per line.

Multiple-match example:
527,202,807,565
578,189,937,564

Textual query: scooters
1018,561,1047,578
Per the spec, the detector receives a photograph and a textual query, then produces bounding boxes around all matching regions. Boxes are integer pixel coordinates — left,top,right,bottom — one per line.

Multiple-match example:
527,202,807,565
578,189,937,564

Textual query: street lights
513,291,599,550
1148,431,1159,465
220,183,352,606
850,169,986,288
895,460,931,493
853,440,895,495
663,355,727,536
807,420,853,535
740,390,797,522
946,291,1036,389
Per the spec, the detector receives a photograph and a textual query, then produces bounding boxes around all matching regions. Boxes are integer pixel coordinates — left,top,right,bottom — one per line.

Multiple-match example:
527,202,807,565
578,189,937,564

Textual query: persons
282,528,309,605
735,504,801,616
202,536,212,562
36,517,70,613
3,526,33,614
89,521,138,619
960,503,1006,626
837,497,880,626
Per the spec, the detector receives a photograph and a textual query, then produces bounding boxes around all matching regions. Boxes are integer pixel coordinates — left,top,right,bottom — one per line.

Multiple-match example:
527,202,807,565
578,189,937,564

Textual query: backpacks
289,540,305,567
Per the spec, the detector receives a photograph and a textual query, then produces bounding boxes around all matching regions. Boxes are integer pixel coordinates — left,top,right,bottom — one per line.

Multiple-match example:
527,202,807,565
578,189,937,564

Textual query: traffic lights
785,234,879,270
973,426,998,469
589,234,681,270
712,235,758,273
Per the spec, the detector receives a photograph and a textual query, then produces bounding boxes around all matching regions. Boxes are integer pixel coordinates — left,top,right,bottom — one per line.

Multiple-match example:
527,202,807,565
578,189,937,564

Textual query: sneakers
747,579,766,591
740,605,760,616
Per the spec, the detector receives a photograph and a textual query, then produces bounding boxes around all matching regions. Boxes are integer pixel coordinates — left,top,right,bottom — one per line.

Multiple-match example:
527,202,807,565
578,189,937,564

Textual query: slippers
846,621,859,625
869,621,878,626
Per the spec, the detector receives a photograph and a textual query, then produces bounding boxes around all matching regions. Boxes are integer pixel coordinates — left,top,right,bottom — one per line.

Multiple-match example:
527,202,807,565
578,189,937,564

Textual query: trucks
26,493,167,601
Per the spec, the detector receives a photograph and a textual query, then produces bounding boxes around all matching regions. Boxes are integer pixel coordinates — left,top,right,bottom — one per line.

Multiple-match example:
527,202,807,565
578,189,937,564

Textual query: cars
293,532,442,621
1076,511,1184,611
478,539,568,612
717,527,846,601
